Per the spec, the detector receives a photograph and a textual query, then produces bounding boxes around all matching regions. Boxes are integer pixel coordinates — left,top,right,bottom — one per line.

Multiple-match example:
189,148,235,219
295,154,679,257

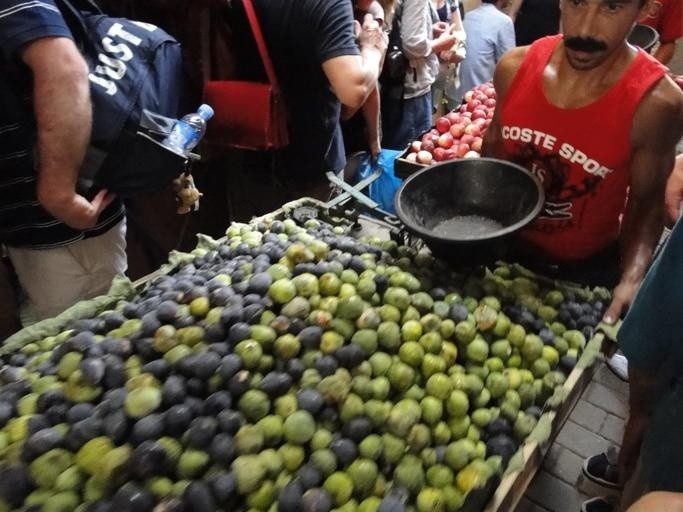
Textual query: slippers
582,495,620,512
583,446,623,489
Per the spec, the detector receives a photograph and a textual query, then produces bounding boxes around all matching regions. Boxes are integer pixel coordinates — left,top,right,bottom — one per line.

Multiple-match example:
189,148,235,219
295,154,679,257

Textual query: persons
346,1,386,164
470,1,683,327
626,1,683,66
579,151,683,512
384,0,458,150
429,1,467,118
444,1,517,113
0,0,131,329
202,0,390,224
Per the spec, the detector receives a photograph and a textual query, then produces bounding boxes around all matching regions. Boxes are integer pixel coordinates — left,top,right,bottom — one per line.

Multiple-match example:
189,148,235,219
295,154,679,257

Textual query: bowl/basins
393,156,545,274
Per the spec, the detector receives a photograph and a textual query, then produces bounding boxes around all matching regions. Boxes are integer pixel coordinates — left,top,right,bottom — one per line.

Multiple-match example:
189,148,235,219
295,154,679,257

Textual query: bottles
160,103,216,156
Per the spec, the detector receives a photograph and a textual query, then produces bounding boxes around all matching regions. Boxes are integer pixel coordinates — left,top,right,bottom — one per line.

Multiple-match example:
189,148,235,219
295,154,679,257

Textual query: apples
405,74,499,164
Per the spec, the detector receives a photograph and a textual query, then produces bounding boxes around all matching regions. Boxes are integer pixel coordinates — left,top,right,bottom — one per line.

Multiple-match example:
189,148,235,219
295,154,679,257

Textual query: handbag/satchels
202,80,289,150
77,13,181,194
378,31,407,87
433,60,460,91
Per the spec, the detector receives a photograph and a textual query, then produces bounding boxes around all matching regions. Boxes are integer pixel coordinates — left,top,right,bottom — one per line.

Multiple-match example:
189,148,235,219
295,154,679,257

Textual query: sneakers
607,354,630,382
